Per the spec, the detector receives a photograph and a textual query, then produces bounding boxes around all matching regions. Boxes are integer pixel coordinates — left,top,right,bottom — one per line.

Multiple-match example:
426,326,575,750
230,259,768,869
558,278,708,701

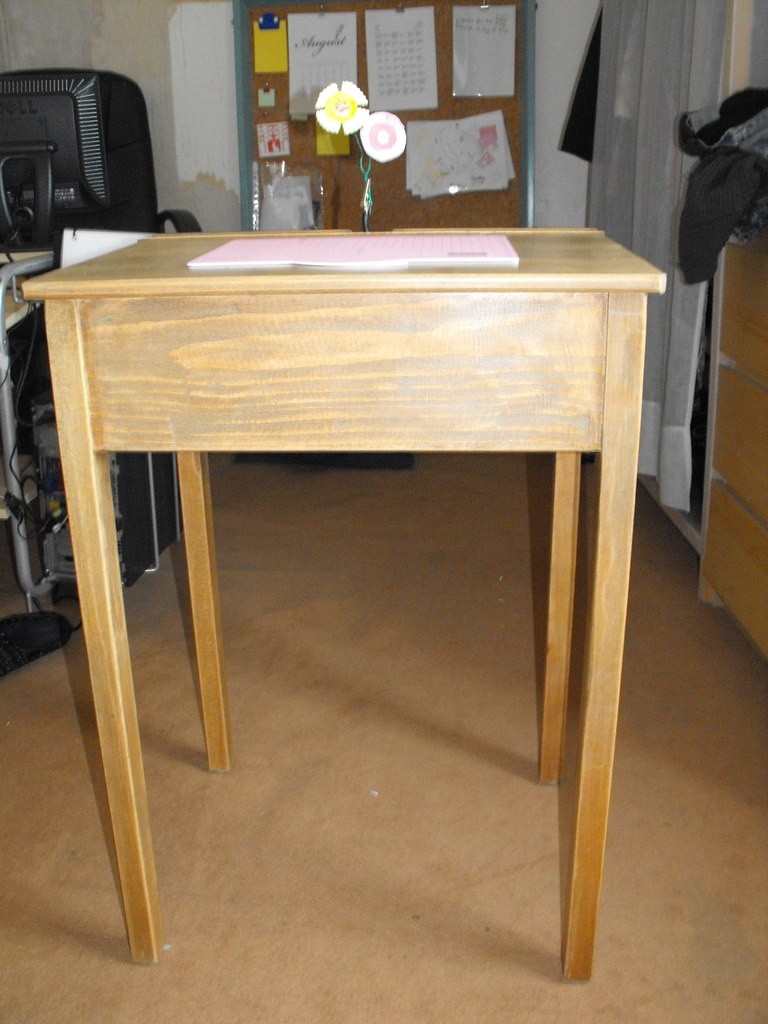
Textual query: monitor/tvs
0,73,111,253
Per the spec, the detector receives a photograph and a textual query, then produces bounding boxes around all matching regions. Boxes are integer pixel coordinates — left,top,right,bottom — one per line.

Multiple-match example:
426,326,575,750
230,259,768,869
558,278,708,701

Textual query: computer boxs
30,386,184,588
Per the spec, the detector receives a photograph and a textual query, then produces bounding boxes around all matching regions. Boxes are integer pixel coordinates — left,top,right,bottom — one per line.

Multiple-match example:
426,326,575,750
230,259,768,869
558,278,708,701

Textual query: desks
23,227,667,982
0,251,61,610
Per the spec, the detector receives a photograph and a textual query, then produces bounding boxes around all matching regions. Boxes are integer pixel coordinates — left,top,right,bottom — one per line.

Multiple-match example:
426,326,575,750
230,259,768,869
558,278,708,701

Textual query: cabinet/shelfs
695,230,768,661
